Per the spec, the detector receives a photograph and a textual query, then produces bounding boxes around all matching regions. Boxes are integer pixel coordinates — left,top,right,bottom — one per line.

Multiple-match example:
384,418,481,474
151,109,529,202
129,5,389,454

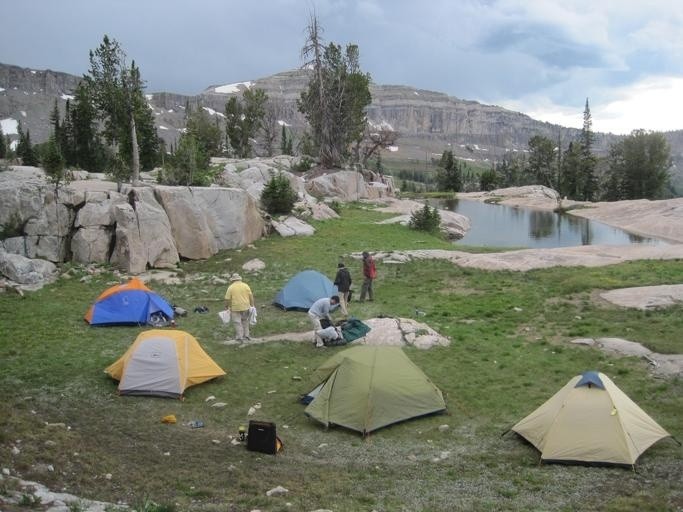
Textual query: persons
308,294,340,348
332,262,352,317
223,273,256,345
356,250,377,304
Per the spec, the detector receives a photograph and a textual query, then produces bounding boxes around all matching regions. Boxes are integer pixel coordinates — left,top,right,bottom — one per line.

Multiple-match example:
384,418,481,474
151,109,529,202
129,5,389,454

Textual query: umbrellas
500,369,681,475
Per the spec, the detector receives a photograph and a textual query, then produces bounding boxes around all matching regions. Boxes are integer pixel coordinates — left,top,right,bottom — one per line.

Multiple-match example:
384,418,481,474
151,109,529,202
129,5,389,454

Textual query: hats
230,273,242,282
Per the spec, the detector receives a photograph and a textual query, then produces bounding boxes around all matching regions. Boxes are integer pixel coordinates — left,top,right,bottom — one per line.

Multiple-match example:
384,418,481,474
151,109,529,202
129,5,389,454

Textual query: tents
104,328,228,401
84,277,176,331
297,342,452,439
271,270,349,313
